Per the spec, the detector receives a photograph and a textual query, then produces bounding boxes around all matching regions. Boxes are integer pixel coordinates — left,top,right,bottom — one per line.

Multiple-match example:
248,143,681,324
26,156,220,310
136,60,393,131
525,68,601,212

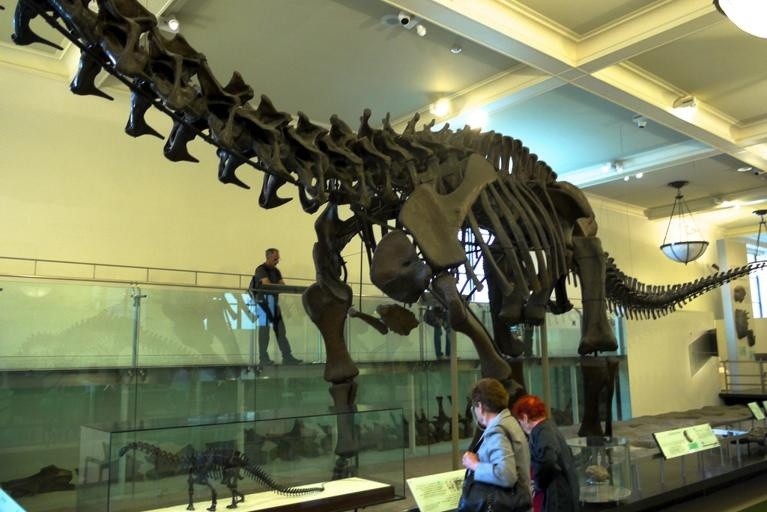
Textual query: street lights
457,424,533,512
248,276,265,303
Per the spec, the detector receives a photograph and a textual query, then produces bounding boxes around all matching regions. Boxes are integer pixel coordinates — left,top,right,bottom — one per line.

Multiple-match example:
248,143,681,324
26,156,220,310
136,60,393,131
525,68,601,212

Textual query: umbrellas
259,355,274,365
281,353,303,365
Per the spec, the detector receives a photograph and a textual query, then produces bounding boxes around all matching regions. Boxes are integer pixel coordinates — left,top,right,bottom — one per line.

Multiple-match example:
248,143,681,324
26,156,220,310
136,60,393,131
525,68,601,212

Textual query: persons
512,395,580,512
435,326,451,359
462,377,532,512
255,249,302,365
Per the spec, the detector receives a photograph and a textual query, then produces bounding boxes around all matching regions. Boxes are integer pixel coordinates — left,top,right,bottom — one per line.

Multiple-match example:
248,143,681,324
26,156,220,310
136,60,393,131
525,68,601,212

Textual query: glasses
659,179,709,265
